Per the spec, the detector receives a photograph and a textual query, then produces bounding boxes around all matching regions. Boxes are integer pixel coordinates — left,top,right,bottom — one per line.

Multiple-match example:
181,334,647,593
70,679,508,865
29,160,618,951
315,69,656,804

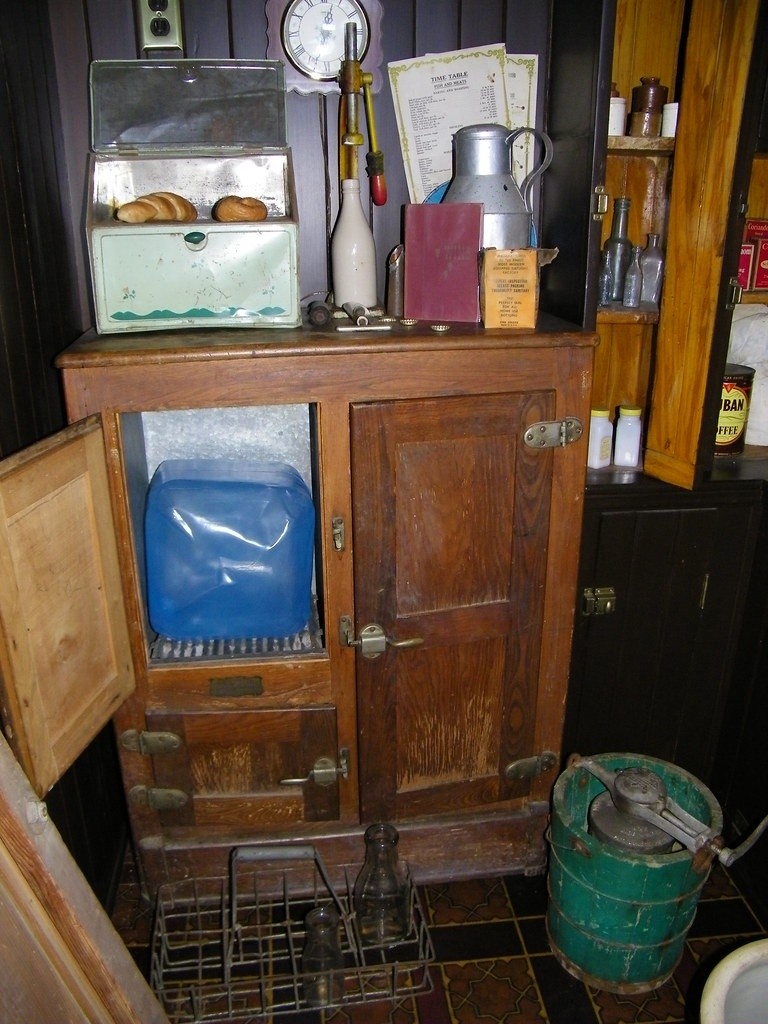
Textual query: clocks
281,0,371,82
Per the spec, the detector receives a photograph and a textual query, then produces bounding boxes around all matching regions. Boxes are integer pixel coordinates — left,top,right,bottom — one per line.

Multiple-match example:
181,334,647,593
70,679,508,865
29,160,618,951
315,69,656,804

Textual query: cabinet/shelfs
2,0,768,900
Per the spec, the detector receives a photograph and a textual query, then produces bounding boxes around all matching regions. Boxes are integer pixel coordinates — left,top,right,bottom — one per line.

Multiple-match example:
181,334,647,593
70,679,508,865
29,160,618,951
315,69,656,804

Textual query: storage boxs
82,57,299,335
737,219,768,292
478,246,558,329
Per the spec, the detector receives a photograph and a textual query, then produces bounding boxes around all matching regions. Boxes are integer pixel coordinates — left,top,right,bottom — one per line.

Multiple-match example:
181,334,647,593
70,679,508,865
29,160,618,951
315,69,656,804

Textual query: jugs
441,122,554,250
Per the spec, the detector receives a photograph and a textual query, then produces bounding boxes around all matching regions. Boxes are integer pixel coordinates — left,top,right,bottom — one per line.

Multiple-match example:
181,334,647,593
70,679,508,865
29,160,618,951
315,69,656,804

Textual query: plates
422,181,538,250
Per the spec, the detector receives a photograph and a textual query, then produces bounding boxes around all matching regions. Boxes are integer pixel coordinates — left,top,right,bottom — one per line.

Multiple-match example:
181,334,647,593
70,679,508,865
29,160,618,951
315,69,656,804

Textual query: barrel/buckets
713,363,756,455
543,753,722,996
699,938,768,1024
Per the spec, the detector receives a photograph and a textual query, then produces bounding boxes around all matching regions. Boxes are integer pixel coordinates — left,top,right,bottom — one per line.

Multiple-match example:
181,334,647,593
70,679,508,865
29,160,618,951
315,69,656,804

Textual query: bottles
640,234,664,308
354,823,410,946
587,406,613,470
599,250,615,307
604,198,633,301
332,177,377,309
614,405,642,467
302,908,344,1010
623,247,643,308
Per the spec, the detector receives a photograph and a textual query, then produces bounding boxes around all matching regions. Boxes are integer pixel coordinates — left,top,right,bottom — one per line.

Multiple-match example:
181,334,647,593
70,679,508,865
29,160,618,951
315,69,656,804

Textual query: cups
606,75,679,138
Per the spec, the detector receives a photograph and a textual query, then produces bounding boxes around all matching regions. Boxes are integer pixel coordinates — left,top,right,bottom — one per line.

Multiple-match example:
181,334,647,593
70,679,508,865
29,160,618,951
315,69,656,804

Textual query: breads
214,196,268,222
116,192,198,223
